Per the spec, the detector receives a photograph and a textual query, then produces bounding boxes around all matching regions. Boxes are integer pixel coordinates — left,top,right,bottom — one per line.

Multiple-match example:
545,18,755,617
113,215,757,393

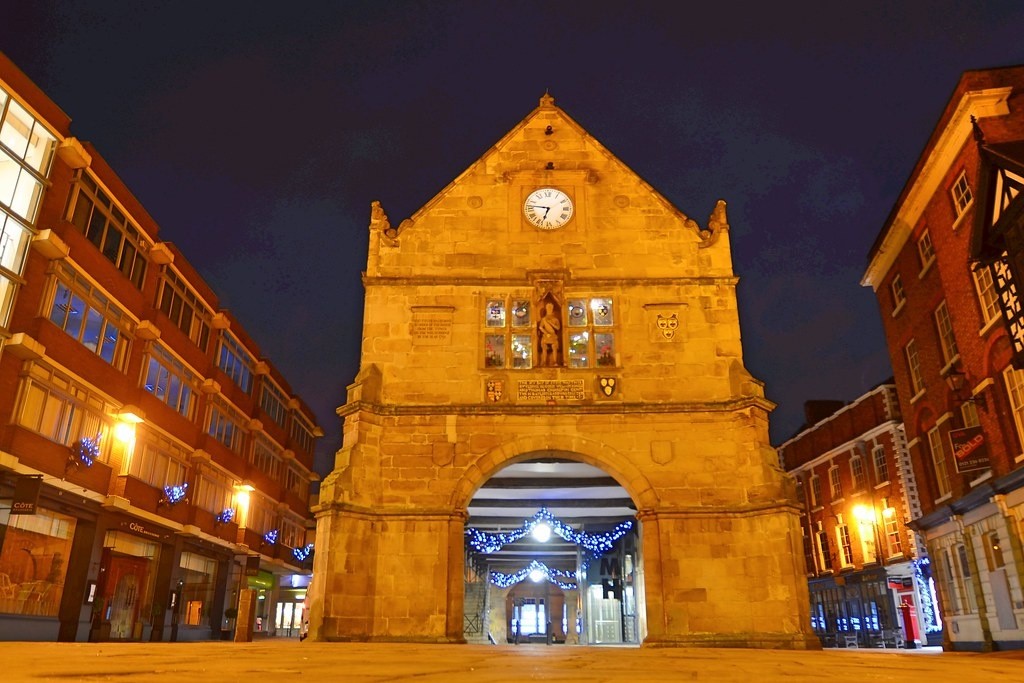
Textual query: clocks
522,187,574,229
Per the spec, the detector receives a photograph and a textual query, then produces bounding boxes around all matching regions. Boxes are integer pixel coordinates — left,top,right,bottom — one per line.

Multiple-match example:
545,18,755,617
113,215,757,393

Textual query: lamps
943,364,988,414
242,478,256,491
120,403,146,423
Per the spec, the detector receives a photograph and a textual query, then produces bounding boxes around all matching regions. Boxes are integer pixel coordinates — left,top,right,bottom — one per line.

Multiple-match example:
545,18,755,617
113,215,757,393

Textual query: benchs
870,627,905,649
826,630,860,648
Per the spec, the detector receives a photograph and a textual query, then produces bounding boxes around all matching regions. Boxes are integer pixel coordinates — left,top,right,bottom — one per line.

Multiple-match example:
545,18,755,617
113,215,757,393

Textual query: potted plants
150,606,163,642
220,608,237,641
89,595,105,641
